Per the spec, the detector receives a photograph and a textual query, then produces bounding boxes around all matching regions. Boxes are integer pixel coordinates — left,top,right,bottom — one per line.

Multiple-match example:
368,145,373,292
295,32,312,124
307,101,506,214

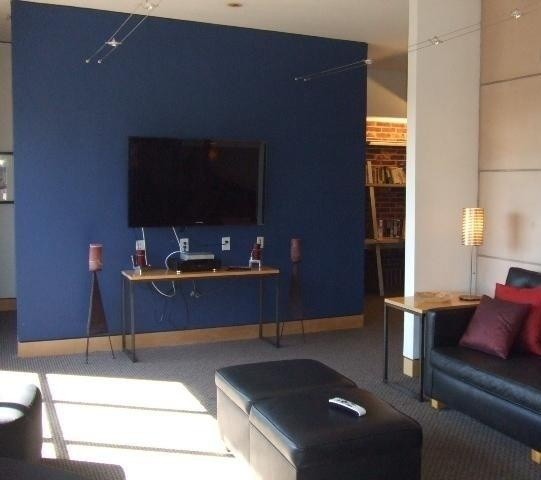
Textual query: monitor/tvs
127,136,265,227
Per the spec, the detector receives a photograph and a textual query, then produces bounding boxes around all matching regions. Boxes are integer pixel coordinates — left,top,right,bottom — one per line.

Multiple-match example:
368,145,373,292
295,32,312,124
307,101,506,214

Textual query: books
365,159,405,241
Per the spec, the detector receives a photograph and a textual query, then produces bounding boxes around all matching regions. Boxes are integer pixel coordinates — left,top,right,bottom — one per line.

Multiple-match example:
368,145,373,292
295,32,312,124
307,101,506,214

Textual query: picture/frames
0,153,16,205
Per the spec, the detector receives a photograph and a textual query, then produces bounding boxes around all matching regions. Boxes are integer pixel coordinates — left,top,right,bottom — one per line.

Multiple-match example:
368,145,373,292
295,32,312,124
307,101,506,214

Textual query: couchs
421,265,541,461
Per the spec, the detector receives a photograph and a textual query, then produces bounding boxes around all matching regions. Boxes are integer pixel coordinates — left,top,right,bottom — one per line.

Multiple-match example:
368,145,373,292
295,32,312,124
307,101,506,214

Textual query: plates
414,292,453,302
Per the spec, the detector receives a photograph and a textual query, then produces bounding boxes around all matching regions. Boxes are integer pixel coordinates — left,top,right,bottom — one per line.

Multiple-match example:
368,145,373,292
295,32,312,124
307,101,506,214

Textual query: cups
133,265,142,276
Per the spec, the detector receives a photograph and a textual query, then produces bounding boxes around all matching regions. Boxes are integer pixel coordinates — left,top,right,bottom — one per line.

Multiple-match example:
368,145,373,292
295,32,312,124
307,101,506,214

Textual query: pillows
494,281,541,355
460,293,530,361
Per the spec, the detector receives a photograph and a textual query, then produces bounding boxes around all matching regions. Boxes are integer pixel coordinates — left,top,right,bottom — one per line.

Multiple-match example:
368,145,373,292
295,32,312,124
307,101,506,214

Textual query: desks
380,289,491,401
122,265,281,362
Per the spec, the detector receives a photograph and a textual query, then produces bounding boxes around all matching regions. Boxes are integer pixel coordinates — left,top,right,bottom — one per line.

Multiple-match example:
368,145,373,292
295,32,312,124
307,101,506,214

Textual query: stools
211,356,425,479
1,384,44,466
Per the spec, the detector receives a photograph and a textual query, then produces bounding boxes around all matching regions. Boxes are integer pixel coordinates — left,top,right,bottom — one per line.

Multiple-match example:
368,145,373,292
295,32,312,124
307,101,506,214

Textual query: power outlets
180,238,189,253
222,236,230,251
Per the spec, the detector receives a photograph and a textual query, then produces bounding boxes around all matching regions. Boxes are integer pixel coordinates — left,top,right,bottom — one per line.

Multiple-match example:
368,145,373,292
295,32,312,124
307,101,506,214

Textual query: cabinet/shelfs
365,138,406,294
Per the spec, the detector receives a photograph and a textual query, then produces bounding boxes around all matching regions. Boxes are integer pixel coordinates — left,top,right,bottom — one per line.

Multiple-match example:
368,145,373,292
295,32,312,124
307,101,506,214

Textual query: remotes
329,397,366,417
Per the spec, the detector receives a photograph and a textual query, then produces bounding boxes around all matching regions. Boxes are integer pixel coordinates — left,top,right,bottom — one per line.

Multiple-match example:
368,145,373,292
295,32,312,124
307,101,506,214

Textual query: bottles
377,220,384,240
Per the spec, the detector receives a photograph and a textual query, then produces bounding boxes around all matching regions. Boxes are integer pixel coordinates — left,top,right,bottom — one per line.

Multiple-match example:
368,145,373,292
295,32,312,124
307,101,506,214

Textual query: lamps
461,205,489,301
280,236,310,338
82,240,118,363
